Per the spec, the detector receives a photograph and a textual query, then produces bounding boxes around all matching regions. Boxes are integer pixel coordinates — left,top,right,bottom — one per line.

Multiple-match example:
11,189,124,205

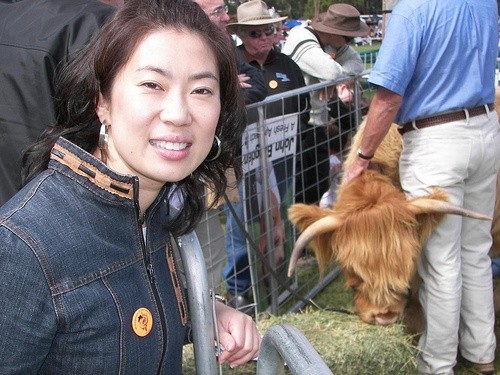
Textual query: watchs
357,150,375,160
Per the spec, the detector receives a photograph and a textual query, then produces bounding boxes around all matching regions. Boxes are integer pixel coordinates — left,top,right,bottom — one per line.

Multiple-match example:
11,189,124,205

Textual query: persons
339,0,500,375
0,0,398,313
0,1,263,375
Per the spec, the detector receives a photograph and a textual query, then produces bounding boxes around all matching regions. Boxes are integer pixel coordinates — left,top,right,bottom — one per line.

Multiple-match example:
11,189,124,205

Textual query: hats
224,0,288,35
311,3,370,37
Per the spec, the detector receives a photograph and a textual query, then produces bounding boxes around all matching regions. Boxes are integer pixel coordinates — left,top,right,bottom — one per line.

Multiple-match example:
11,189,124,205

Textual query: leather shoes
226,292,256,317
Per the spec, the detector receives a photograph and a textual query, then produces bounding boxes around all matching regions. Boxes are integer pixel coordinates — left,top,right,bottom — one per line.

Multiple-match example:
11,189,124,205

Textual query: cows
287,104,499,345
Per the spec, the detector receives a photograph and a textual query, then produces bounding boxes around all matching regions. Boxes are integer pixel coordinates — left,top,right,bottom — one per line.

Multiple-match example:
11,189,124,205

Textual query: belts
398,103,495,136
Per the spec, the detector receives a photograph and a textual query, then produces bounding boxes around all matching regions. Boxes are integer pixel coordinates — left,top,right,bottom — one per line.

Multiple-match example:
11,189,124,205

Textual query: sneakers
296,255,319,267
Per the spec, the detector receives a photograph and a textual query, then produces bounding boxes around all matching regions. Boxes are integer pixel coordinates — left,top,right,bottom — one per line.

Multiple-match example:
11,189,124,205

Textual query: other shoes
465,361,496,372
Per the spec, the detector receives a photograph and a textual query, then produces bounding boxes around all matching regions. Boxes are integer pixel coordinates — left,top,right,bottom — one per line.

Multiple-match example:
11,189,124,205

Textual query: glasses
341,35,354,43
208,6,229,17
241,26,274,38
